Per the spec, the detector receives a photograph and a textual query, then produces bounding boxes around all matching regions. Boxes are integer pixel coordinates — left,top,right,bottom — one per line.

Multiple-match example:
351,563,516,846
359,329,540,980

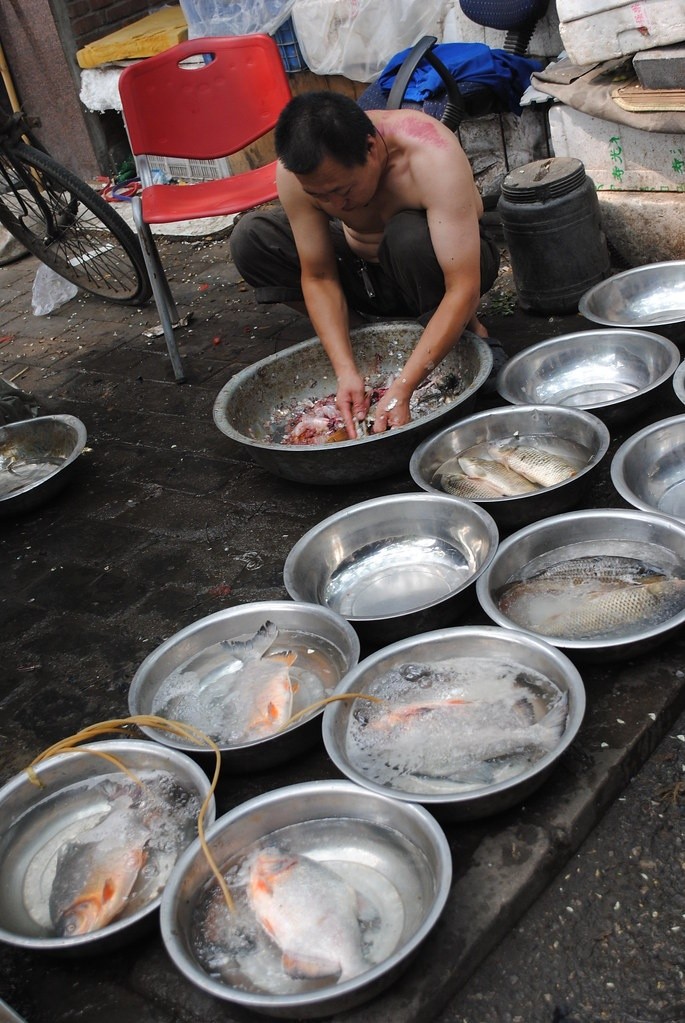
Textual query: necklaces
364,123,391,209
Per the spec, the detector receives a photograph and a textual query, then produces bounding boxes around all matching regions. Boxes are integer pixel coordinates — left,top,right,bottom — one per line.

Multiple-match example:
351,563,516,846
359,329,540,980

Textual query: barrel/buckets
497,156,612,317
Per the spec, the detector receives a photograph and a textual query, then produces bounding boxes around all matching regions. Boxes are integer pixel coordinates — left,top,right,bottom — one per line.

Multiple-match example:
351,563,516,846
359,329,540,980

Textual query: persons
230,88,517,442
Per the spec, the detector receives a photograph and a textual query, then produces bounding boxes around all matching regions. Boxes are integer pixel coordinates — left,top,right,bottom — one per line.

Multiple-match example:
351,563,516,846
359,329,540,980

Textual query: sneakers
479,335,512,397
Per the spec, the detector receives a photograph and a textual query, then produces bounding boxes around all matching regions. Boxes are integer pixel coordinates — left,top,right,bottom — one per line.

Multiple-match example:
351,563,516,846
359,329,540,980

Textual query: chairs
115,33,295,388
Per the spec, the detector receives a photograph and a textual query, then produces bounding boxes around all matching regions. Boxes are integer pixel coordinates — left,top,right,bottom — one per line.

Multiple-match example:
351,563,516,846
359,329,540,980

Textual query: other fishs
48,780,163,944
440,442,583,501
499,552,685,643
365,689,570,785
247,846,384,986
285,372,459,446
207,619,298,747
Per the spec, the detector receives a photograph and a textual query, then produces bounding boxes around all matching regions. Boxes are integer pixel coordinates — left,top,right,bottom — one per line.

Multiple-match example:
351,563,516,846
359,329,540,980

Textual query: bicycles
1,109,152,309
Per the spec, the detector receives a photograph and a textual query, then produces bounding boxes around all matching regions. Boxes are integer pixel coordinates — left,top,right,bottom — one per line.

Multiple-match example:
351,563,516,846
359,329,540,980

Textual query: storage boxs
122,66,371,188
177,1,309,75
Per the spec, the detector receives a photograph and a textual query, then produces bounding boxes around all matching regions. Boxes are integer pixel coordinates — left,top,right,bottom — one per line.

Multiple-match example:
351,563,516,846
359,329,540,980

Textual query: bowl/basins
408,406,609,527
673,357,684,406
579,259,685,329
0,414,87,517
159,780,454,1020
609,412,685,526
495,328,682,427
282,489,501,634
126,599,359,776
210,321,496,487
476,509,685,664
321,625,589,820
1,737,219,948
